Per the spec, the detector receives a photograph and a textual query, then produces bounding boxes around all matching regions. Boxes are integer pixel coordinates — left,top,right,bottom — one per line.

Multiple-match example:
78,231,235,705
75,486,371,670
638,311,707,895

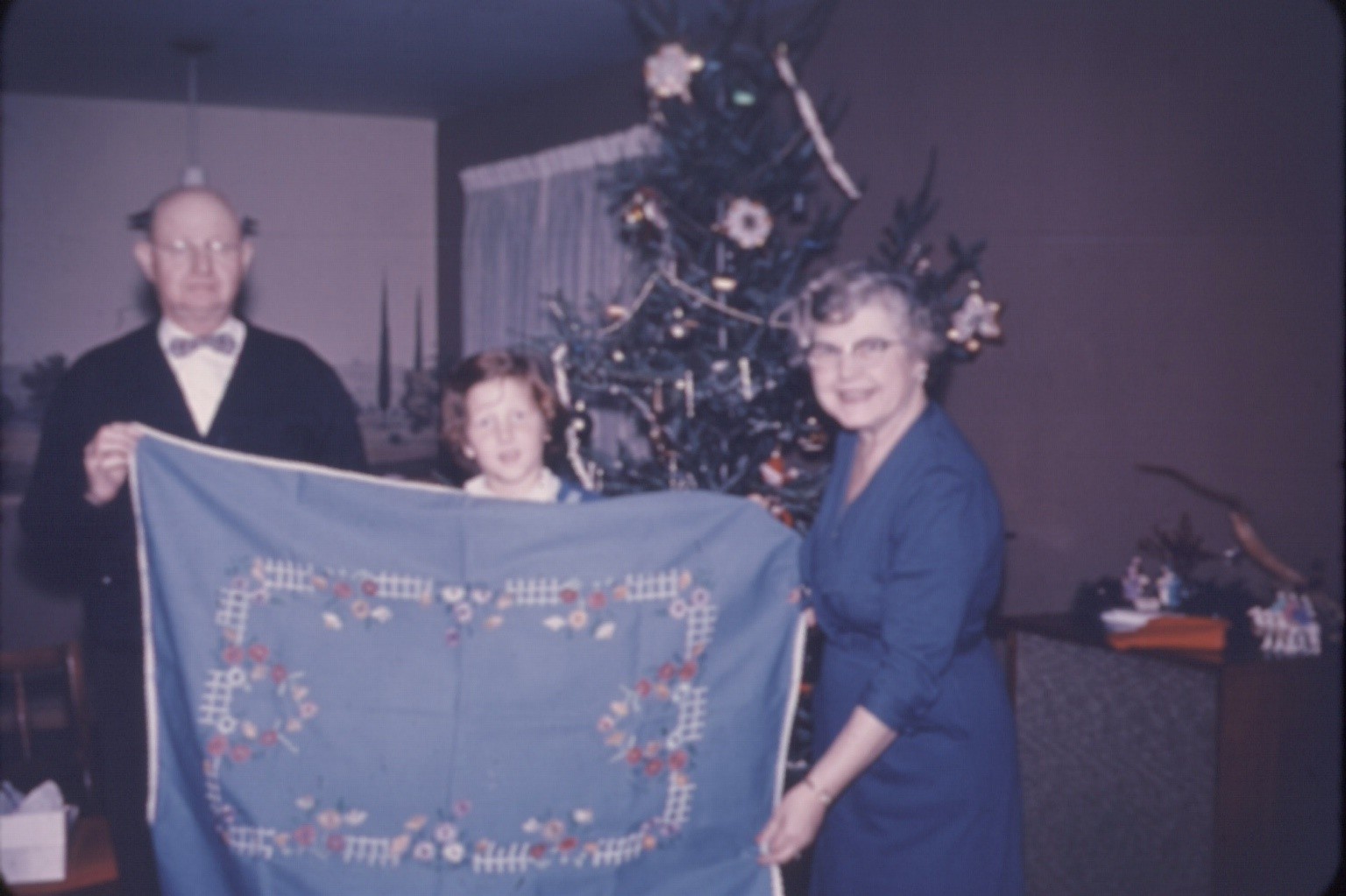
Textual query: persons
757,243,1026,896
439,348,608,507
23,185,364,896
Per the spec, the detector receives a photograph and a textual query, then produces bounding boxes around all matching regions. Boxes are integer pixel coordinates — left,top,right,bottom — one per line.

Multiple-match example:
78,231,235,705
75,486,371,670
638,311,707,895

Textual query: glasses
151,239,248,261
808,329,916,368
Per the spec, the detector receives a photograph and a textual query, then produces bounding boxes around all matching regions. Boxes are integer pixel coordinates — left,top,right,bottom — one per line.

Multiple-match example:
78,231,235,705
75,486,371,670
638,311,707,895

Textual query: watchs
802,776,835,804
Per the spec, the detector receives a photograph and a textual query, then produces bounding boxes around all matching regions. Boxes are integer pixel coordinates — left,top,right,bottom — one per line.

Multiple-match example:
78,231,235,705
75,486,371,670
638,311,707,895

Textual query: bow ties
170,334,237,356
